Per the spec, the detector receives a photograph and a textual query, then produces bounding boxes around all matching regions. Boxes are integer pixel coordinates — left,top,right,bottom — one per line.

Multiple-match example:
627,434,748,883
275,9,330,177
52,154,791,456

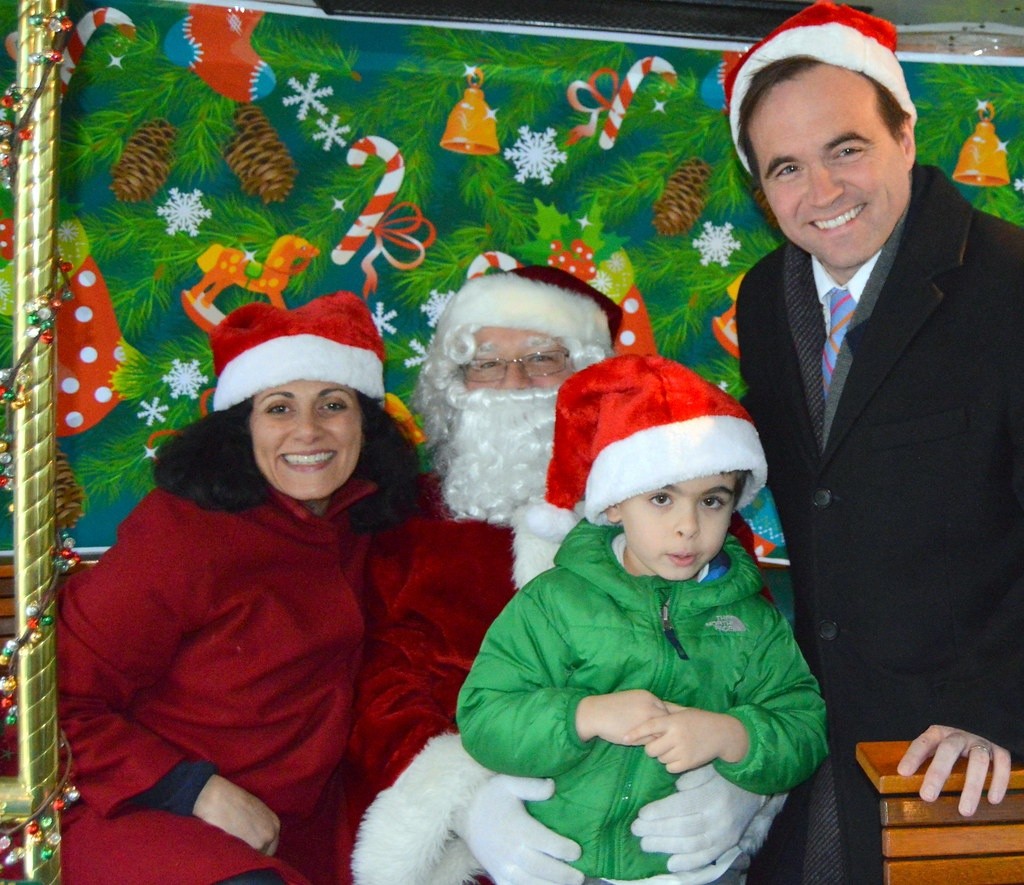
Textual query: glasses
460,349,567,382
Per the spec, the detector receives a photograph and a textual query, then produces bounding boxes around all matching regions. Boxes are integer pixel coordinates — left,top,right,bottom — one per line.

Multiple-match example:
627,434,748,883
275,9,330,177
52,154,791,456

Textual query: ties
822,289,858,403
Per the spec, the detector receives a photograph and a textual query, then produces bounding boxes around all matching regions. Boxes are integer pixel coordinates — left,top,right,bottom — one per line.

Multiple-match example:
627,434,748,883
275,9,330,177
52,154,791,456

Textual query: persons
325,263,794,884
456,354,828,884
49,292,451,884
723,0,1023,884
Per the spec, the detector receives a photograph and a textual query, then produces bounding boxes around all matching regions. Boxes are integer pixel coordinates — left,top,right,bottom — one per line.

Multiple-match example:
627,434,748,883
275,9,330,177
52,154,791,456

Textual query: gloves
632,762,768,871
449,776,587,885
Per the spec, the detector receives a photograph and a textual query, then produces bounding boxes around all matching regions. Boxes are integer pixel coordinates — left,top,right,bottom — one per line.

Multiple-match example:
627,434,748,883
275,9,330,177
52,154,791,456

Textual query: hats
723,0,917,175
436,265,624,362
207,292,383,407
552,353,769,528
